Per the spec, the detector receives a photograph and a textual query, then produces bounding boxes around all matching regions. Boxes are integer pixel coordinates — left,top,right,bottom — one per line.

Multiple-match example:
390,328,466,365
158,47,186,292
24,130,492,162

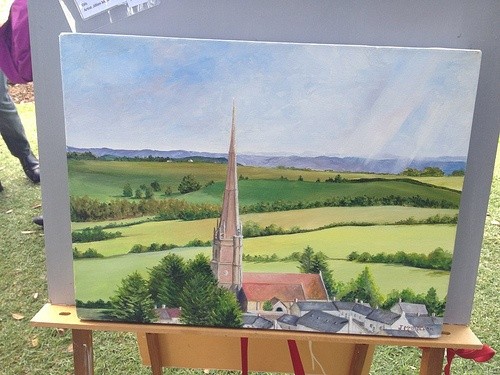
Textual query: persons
0,68,41,193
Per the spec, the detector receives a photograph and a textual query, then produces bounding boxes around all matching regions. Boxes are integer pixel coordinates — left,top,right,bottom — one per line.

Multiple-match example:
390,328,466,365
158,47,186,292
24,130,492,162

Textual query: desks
29,304,483,374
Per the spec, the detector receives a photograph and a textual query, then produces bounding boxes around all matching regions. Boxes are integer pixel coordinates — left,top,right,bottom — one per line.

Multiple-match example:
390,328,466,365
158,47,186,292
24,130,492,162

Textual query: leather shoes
20,152,40,182
